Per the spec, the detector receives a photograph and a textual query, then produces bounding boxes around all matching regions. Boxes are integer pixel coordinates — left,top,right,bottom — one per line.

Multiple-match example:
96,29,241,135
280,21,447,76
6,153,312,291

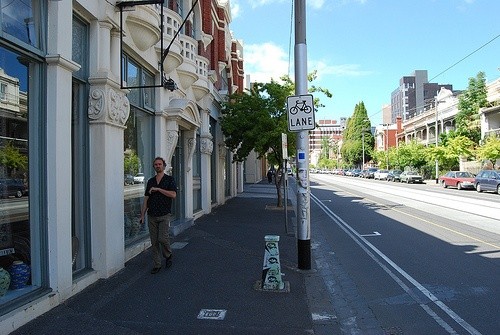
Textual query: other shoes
151,267,161,274
165,257,171,269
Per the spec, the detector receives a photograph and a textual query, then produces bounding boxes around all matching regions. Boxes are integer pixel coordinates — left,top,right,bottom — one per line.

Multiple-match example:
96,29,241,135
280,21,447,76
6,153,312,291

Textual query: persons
267,170,273,185
140,157,177,274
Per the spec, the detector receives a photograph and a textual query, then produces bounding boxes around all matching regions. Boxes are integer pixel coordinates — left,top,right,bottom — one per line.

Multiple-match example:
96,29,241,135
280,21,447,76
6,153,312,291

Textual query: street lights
363,119,389,171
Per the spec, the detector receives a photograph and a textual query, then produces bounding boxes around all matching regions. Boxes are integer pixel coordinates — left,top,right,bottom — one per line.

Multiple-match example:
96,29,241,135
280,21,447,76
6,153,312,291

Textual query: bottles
8,261,30,290
0,267,11,297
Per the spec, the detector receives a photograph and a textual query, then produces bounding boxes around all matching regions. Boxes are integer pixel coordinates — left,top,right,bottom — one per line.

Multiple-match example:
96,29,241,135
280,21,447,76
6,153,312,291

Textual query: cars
133,172,145,184
438,170,476,190
287,169,293,177
309,167,378,179
0,177,29,199
385,169,403,182
373,169,389,180
124,173,135,186
473,169,500,195
399,170,424,184
